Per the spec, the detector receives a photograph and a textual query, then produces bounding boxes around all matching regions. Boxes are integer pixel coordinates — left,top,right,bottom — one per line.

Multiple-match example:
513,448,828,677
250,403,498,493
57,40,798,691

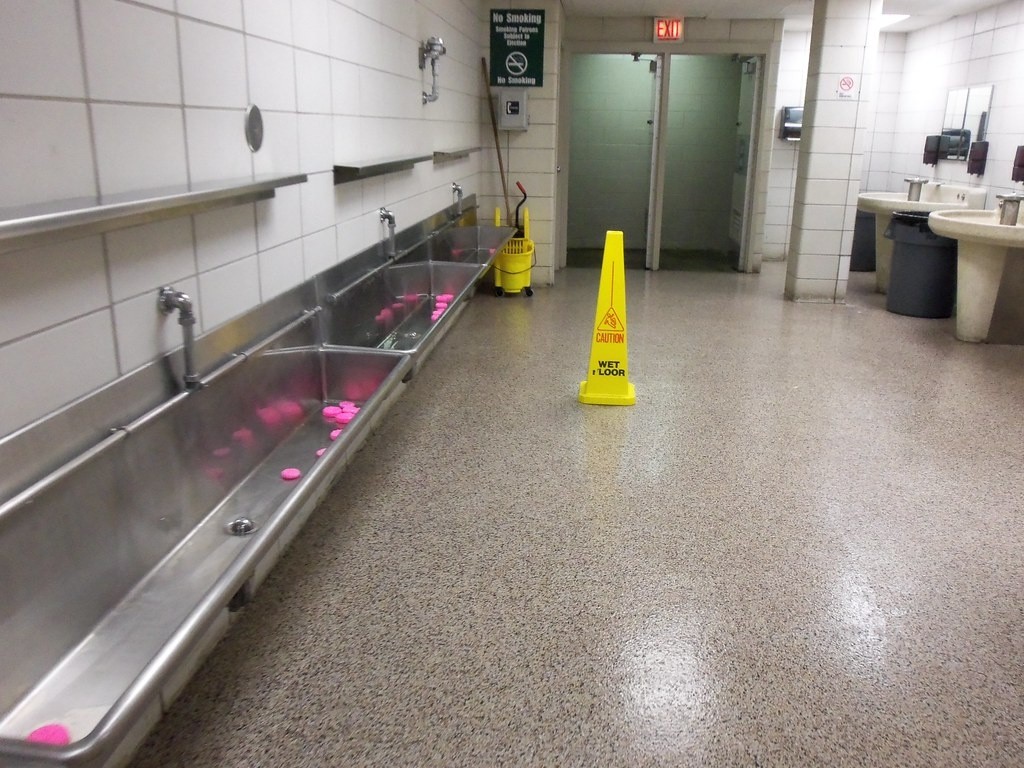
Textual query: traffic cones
579,231,636,407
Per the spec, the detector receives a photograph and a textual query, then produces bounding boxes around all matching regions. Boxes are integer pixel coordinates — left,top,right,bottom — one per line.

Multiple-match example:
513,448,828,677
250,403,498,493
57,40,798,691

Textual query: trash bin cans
883,210,958,318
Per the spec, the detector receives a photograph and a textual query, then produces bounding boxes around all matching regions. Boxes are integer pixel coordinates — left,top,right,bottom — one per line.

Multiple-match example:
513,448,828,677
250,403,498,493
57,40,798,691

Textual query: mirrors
938,84,970,160
958,84,994,162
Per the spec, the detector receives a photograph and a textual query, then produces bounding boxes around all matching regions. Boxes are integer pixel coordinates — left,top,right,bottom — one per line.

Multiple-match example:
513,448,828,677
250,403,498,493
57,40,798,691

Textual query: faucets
995,194,1024,226
903,177,929,202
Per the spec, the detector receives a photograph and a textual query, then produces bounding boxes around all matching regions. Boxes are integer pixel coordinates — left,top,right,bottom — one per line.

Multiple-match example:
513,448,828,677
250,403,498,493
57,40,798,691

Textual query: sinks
391,225,516,264
857,192,966,213
259,262,485,350
1,351,404,768
928,209,1024,249
